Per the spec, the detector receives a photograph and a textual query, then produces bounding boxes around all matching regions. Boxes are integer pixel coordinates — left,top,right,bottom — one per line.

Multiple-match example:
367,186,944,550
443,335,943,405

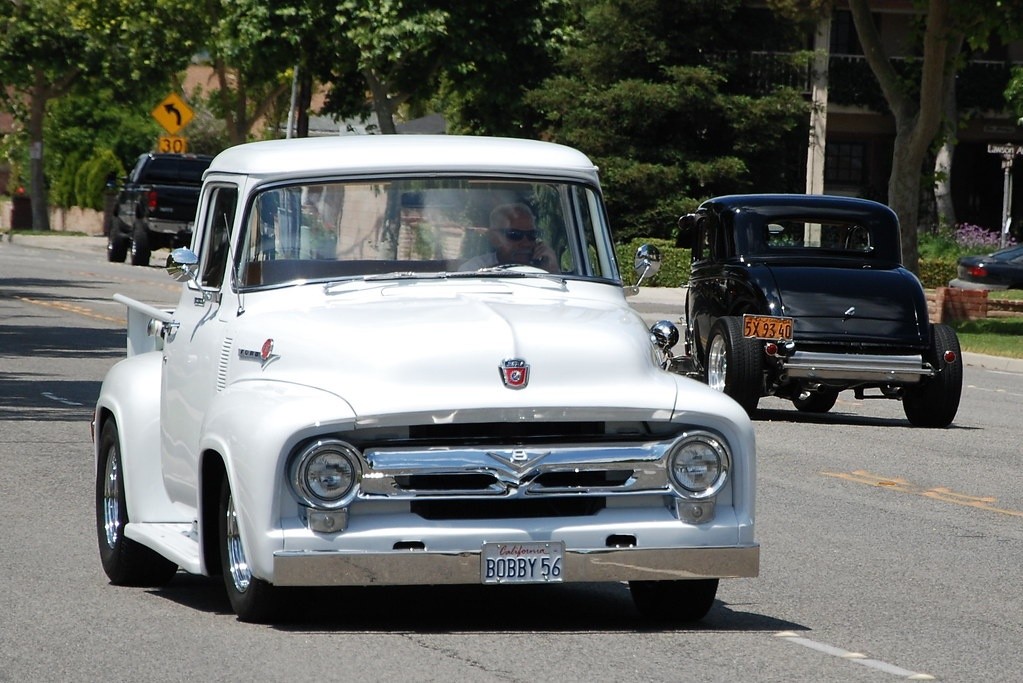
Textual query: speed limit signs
157,136,187,156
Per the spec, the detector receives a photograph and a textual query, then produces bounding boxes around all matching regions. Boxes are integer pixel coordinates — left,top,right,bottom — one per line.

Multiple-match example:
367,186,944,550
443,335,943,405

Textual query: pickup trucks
90,131,761,628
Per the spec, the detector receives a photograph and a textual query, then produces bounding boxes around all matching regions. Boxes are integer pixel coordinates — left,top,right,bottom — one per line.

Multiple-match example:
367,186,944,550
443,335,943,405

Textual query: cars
948,241,1023,295
671,192,965,429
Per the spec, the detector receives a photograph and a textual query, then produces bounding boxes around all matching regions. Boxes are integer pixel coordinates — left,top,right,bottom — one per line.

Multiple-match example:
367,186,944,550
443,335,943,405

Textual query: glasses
491,228,540,241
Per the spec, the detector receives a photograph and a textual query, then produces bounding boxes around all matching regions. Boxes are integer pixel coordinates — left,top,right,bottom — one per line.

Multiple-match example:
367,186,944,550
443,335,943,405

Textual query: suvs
101,149,218,266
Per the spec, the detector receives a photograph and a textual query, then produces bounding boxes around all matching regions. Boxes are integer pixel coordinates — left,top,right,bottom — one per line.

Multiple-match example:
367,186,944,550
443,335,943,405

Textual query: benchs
247,259,467,285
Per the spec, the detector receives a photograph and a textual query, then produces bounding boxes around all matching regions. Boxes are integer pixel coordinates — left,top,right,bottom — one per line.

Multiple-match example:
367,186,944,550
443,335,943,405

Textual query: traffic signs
987,143,1023,157
1000,158,1013,168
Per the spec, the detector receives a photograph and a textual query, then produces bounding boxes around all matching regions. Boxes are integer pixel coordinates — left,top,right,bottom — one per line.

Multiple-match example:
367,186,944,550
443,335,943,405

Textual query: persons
458,203,561,274
